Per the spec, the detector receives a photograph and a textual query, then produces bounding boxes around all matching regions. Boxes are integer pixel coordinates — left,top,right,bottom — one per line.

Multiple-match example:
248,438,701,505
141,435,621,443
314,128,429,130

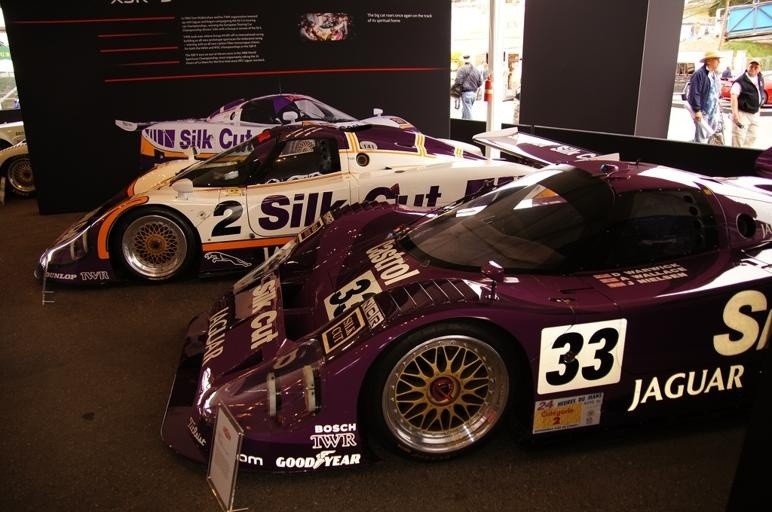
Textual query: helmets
460,53,475,63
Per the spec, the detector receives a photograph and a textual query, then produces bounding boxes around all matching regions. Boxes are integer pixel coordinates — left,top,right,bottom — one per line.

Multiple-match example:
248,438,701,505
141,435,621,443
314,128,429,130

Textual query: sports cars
115,93,423,171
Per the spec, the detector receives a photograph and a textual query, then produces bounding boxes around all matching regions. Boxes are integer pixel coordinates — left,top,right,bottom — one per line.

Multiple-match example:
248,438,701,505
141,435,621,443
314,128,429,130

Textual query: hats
749,58,762,66
698,52,723,62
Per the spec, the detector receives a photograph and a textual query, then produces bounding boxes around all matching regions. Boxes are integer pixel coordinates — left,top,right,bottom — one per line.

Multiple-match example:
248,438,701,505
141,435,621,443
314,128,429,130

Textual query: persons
722,66,732,77
508,53,523,125
687,51,726,145
730,56,763,147
453,52,484,122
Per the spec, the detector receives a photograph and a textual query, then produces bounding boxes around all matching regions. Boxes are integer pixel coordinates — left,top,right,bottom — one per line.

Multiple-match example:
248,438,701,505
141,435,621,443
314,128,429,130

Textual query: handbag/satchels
449,84,462,99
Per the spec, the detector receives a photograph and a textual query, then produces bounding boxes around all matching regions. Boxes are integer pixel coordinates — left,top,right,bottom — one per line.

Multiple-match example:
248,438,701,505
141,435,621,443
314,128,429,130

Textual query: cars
14,95,21,110
0,121,36,198
721,71,772,107
